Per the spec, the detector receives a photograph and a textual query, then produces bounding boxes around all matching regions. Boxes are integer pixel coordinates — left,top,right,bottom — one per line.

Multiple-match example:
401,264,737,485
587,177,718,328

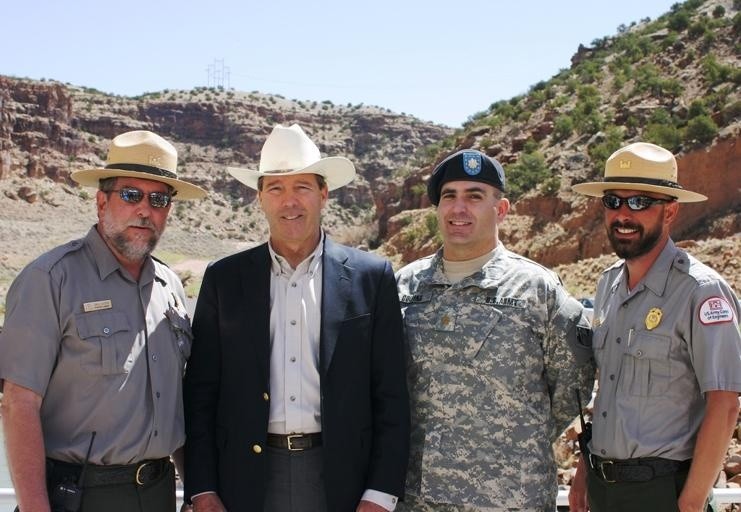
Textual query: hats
71,130,206,200
228,124,356,191
428,150,505,206
570,143,709,203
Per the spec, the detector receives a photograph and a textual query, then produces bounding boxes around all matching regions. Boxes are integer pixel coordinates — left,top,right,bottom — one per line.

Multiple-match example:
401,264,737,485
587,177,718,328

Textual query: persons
399,148,593,512
181,124,408,512
0,128,208,512
565,141,741,512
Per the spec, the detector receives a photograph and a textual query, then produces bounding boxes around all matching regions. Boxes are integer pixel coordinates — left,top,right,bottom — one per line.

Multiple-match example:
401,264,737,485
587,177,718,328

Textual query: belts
583,449,691,483
265,433,321,452
83,457,171,487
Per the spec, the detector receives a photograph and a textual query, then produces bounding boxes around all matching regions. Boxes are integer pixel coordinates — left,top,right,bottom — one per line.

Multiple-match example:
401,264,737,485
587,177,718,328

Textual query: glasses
109,186,171,208
602,194,665,211
